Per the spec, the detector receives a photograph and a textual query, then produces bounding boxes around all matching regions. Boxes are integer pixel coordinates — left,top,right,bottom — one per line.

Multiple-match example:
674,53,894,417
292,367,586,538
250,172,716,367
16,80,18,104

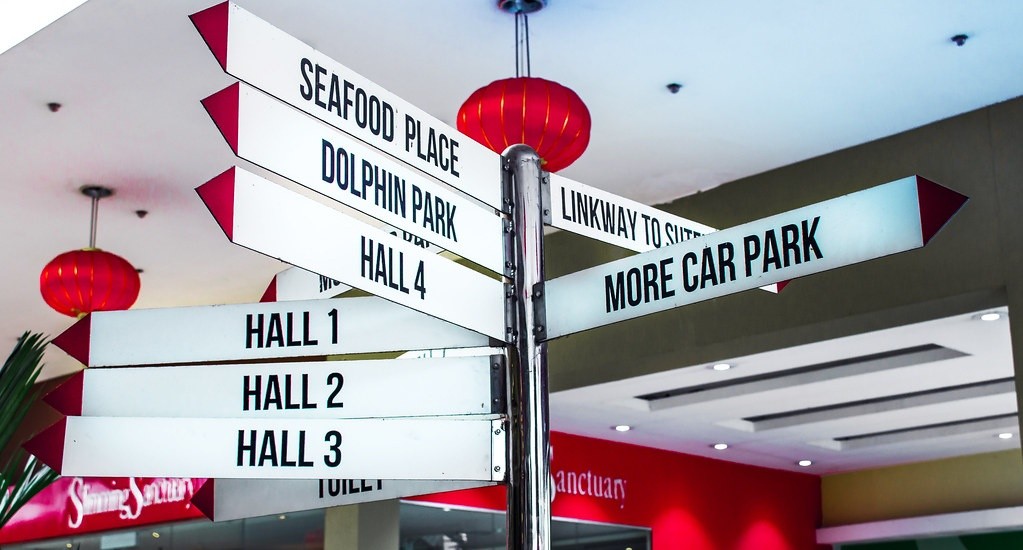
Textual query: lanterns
38,248,142,320
454,76,592,174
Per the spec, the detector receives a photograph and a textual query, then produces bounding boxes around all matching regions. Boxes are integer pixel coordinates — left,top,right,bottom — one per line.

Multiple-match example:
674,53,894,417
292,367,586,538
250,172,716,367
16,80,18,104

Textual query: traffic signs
189,479,506,522
530,174,973,346
200,81,514,280
194,164,513,344
540,170,793,295
50,298,509,368
187,1,510,217
260,224,447,303
19,414,507,482
39,353,508,418
